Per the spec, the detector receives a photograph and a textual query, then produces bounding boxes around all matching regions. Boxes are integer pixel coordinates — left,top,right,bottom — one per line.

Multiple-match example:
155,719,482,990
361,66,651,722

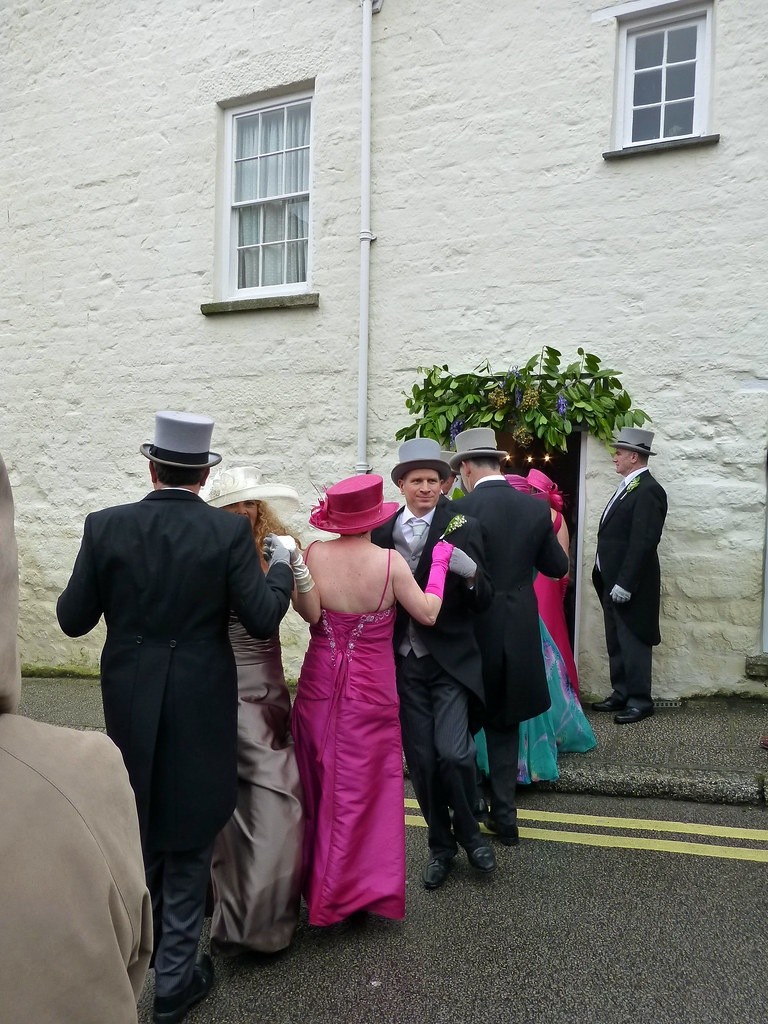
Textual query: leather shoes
614,705,654,723
592,697,626,711
454,830,497,873
484,811,519,845
422,856,453,889
471,796,488,821
154,953,215,1024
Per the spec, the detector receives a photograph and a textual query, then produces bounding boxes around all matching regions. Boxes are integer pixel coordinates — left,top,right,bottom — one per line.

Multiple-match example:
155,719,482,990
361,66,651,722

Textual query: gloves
425,542,453,601
609,583,631,603
443,539,477,579
277,535,315,593
262,533,290,568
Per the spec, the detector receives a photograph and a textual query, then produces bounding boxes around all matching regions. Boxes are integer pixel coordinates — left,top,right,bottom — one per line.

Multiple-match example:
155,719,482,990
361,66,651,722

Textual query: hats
141,412,222,468
504,474,549,501
449,427,507,474
525,469,563,511
439,450,457,473
308,473,399,535
609,427,657,455
392,439,452,486
205,466,300,524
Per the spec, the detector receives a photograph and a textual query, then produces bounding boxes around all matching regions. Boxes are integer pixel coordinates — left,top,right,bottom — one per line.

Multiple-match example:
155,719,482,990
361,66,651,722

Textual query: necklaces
342,534,369,541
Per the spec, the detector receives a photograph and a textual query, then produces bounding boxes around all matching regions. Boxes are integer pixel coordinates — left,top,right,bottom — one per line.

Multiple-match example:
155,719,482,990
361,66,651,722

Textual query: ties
411,521,427,550
601,479,625,524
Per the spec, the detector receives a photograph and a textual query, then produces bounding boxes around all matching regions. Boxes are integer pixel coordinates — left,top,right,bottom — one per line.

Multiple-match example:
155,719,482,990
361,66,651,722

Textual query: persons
371,437,498,890
591,424,668,724
205,465,320,956
441,452,458,495
292,473,453,926
448,427,568,845
474,468,599,785
0,453,154,1024
57,411,295,1024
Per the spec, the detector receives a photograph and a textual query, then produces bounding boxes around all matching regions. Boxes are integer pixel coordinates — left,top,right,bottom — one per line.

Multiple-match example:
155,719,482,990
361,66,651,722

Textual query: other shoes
759,736,768,748
